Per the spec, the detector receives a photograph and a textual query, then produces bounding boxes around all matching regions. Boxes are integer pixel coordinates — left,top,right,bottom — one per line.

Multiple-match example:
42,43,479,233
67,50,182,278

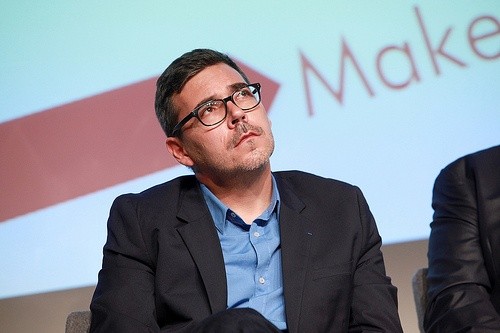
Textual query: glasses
172,83,262,136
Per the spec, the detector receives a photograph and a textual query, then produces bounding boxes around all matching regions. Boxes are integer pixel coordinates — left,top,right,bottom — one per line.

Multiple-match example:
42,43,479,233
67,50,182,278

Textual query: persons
424,145,500,333
85,49,403,333
